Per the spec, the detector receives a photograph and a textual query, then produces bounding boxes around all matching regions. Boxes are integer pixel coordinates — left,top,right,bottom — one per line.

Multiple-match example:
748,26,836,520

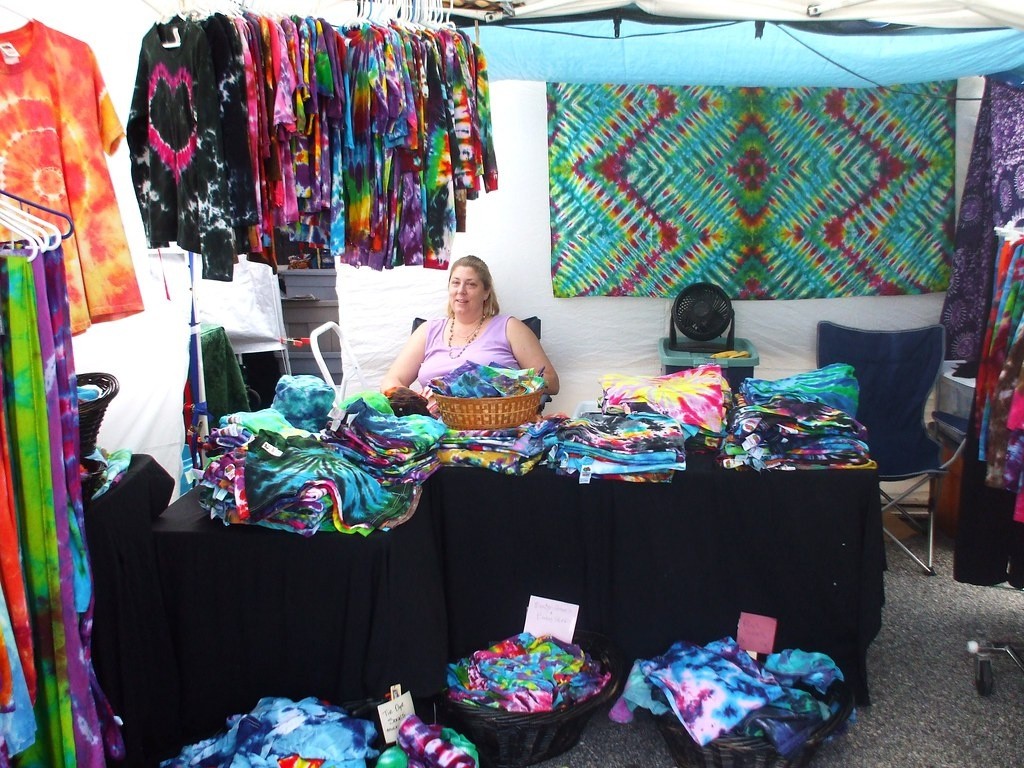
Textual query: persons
379,255,560,395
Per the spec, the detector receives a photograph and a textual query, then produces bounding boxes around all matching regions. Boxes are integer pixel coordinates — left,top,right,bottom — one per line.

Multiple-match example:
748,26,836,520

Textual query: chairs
412,317,542,347
814,319,950,576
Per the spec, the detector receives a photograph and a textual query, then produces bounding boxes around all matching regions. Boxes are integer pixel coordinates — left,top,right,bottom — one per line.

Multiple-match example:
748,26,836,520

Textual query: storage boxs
272,269,343,386
924,422,967,539
660,336,759,394
931,359,976,446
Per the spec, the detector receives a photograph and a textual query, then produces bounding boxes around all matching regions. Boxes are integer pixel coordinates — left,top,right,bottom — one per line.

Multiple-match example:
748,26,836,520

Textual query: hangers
159,0,456,32
0,188,74,263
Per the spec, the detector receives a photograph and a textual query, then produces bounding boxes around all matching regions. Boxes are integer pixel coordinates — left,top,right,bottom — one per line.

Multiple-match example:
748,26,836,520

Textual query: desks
149,440,885,710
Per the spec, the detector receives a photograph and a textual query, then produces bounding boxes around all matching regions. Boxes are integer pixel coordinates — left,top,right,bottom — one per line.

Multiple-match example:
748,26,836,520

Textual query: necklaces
456,324,479,342
449,313,486,359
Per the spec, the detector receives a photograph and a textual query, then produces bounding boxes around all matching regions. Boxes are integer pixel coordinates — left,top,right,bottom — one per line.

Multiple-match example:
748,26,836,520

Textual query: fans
669,281,736,353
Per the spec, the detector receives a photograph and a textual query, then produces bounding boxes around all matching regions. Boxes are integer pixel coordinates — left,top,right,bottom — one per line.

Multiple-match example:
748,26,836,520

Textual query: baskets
76,373,120,457
438,630,622,768
78,457,106,510
646,650,855,768
434,386,546,430
